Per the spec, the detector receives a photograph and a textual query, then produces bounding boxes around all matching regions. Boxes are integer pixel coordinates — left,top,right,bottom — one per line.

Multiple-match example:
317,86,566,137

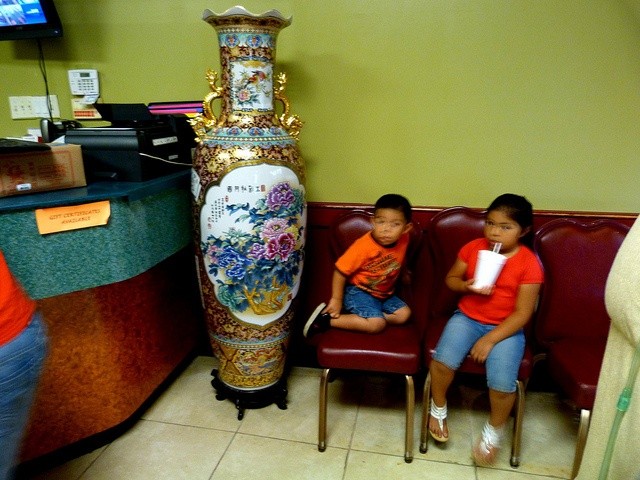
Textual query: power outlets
8,96,33,120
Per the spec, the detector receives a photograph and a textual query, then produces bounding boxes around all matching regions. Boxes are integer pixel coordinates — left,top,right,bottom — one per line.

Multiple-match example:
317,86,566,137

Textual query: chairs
419,204,541,470
315,211,421,465
533,220,632,480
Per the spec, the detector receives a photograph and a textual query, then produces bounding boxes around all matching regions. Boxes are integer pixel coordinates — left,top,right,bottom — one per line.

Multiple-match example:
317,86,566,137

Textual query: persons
0,251,49,480
428,193,545,465
303,194,414,338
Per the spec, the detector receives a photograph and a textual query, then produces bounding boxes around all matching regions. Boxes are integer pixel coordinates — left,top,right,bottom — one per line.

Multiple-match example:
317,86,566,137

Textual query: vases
184,4,307,391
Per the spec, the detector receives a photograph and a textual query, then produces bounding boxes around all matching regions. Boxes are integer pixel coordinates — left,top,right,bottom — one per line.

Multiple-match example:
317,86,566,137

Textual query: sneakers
302,303,330,338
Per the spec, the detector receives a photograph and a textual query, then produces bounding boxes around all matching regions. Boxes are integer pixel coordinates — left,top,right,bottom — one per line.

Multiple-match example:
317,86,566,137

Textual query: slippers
427,398,452,444
471,420,508,470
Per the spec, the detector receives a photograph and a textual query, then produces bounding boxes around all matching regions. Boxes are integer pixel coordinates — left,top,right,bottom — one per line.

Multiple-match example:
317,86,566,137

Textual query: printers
64,101,195,181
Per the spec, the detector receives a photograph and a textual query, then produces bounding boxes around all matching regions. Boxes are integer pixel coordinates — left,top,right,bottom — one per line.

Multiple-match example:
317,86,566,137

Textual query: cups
472,250,508,290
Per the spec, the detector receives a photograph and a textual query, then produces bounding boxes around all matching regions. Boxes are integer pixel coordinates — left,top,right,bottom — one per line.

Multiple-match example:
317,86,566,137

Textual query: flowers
201,182,304,311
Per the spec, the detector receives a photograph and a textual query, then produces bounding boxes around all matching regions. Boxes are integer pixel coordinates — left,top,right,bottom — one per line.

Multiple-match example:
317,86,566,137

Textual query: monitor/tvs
0,0,66,45
